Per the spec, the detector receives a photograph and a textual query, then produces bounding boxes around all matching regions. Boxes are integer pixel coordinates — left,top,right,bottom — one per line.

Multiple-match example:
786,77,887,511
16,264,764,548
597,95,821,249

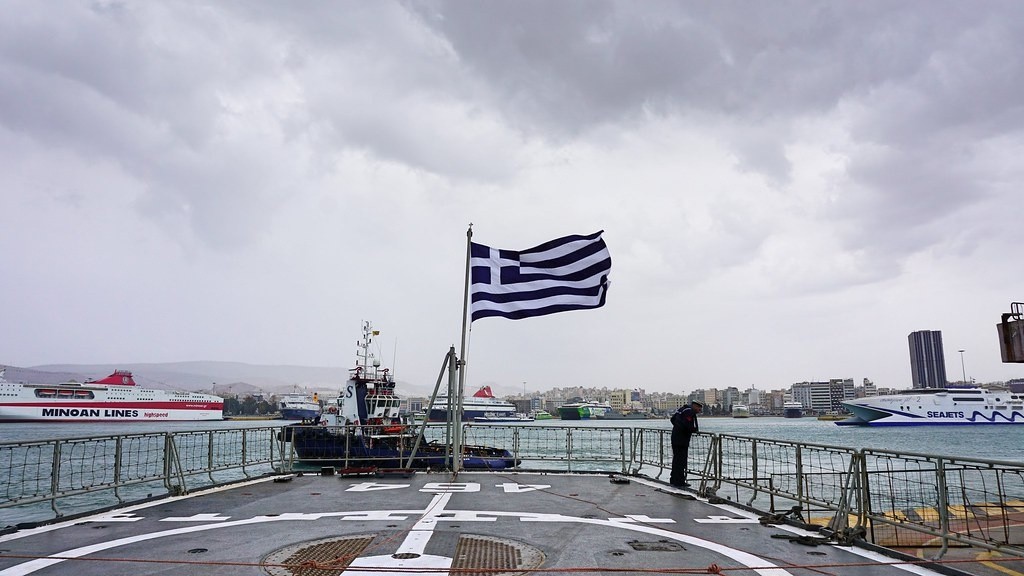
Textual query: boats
528,409,552,419
595,402,651,420
0,369,225,423
834,418,865,426
557,396,612,420
323,396,337,414
278,385,320,419
421,383,518,421
840,385,1024,426
817,416,846,420
277,321,523,469
472,416,535,423
732,405,749,418
516,413,524,418
784,400,803,418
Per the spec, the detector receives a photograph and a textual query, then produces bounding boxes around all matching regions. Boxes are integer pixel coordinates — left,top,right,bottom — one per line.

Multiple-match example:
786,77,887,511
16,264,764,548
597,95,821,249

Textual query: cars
851,413,855,417
847,413,852,416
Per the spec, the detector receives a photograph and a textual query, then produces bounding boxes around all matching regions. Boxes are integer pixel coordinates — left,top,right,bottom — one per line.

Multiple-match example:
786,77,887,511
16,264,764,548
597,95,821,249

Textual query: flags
469,230,611,323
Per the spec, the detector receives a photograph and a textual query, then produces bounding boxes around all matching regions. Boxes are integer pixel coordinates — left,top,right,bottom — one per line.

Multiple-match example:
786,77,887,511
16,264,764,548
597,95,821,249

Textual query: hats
692,399,704,406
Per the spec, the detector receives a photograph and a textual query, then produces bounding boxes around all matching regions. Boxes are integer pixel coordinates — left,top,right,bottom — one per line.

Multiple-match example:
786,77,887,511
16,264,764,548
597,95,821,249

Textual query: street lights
524,382,526,399
212,382,216,395
958,350,966,383
229,385,232,411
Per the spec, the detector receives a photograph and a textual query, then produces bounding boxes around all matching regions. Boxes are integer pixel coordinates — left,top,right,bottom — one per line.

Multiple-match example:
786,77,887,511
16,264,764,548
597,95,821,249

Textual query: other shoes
670,476,691,487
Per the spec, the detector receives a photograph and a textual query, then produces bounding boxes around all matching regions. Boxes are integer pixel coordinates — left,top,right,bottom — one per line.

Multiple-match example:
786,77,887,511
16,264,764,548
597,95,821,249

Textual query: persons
670,399,704,487
